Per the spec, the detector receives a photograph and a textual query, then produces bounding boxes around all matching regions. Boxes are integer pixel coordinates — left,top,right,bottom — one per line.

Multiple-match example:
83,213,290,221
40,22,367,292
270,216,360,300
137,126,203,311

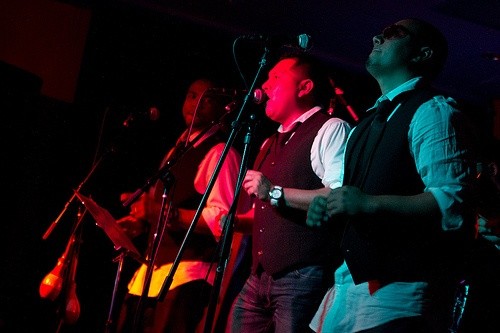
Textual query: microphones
239,33,313,52
124,108,161,127
329,77,359,122
210,87,266,104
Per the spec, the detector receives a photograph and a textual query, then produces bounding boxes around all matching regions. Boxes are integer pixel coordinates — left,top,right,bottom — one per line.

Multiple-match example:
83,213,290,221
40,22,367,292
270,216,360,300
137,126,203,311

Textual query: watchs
269,185,283,207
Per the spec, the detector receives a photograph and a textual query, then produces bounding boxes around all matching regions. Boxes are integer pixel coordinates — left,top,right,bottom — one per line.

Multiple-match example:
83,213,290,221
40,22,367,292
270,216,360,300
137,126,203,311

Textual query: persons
306,17,480,333
115,78,241,333
222,52,351,333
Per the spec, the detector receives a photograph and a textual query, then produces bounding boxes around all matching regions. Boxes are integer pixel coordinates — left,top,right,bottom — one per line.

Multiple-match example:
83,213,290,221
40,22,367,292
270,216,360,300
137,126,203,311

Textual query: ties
351,100,393,188
275,121,302,155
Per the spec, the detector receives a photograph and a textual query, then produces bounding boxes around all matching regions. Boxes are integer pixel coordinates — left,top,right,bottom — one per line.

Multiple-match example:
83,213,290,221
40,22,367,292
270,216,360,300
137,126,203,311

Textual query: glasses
383,24,422,48
